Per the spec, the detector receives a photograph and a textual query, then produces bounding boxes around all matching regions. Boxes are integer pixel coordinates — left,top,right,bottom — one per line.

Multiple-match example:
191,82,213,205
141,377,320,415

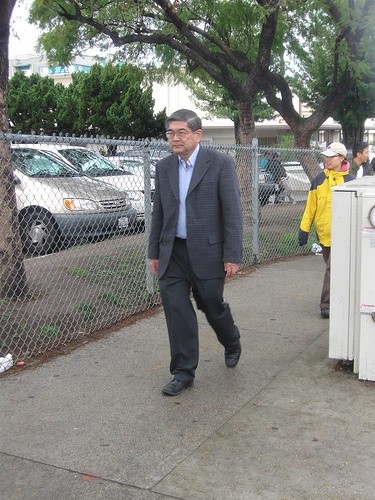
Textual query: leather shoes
224,340,242,368
162,379,192,396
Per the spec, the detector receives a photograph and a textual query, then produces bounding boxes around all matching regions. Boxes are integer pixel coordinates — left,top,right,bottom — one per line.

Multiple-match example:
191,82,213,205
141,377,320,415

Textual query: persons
148,109,242,394
298,142,356,318
261,150,294,203
348,142,375,178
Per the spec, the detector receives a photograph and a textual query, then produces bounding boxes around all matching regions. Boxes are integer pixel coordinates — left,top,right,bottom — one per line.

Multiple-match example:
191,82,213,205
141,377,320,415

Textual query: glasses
166,128,199,139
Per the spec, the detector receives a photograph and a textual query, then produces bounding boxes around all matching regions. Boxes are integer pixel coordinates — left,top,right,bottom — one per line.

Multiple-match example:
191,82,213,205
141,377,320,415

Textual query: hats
320,143,347,157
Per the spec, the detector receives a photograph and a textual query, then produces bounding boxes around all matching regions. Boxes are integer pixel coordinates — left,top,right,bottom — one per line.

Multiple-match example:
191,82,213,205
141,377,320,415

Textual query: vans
10,144,279,255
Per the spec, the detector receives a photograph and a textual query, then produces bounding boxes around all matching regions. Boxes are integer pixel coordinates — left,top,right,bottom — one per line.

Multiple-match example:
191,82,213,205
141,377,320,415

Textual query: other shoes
321,307,329,318
